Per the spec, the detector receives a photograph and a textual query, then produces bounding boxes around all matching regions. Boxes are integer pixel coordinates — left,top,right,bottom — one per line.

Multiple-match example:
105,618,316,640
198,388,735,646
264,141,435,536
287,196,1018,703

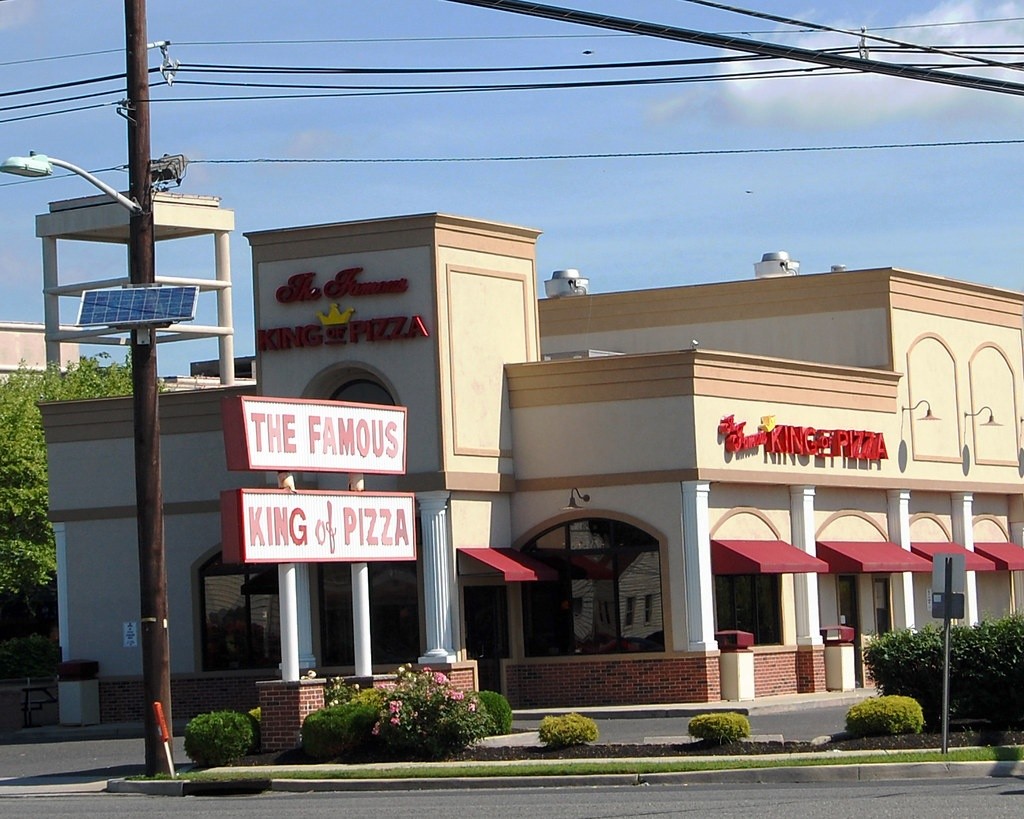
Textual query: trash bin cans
715,630,756,702
57,659,102,726
820,625,857,692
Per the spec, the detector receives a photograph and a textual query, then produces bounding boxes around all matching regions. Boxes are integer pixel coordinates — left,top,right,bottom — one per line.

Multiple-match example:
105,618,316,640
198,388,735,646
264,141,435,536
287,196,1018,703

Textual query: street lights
0,151,176,779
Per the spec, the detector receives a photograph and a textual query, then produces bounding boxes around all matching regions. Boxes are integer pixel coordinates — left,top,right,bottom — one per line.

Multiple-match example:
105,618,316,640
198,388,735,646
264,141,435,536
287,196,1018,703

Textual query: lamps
561,487,589,509
965,406,1001,425
902,400,940,420
